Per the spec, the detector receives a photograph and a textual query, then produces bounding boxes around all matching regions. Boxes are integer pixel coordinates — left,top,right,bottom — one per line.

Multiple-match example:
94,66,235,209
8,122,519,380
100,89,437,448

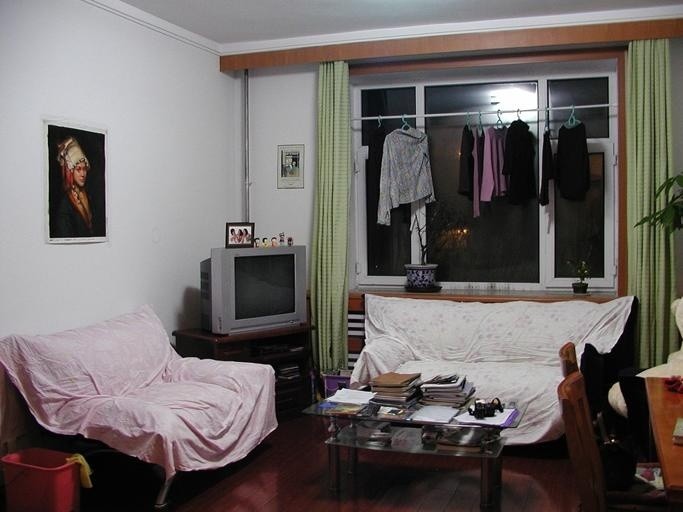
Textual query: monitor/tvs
201,245,306,336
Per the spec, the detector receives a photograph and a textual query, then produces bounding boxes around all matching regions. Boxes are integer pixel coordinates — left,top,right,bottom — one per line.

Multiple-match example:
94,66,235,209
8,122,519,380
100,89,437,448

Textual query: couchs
0,300,281,512
347,290,640,461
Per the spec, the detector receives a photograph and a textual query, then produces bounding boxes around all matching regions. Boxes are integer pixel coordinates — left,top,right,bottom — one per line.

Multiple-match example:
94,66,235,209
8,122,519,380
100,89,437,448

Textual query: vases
402,259,444,294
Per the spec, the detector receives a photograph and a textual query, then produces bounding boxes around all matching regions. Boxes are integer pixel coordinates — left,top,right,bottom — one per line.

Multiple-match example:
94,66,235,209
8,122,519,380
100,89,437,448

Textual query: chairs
555,367,670,511
556,338,578,381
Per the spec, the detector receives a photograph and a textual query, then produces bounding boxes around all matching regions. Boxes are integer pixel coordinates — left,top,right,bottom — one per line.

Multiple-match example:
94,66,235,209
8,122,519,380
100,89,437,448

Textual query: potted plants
564,252,593,294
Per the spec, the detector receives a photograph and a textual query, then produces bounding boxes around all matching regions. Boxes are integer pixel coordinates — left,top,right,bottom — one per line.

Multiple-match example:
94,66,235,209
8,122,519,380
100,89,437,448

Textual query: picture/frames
223,220,255,249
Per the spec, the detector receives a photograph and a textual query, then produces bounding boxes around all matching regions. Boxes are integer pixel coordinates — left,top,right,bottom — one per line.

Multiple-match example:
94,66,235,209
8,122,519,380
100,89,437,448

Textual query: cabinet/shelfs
168,321,322,427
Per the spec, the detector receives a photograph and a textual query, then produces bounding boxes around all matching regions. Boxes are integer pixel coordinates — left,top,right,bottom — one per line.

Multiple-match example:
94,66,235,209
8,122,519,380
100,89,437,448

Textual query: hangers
376,115,384,131
462,104,583,134
398,113,412,131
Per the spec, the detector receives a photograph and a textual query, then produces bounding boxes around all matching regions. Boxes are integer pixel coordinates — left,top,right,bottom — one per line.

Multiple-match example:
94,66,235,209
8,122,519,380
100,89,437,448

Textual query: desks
643,362,683,507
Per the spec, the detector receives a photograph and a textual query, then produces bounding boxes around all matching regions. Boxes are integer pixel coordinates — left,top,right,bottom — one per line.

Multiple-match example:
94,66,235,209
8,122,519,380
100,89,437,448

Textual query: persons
229,229,251,243
55,135,104,236
255,231,293,248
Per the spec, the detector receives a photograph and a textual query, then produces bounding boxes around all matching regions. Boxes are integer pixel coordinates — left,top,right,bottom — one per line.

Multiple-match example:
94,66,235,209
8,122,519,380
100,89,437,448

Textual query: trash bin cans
1,448,80,512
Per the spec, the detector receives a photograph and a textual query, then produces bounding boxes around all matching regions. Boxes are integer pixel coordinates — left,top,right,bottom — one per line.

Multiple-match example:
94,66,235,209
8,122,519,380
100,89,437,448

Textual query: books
355,371,519,453
672,417,683,444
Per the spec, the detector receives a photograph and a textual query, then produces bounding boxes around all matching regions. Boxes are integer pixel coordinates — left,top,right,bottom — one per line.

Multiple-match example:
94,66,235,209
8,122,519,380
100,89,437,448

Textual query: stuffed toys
609,298,683,415
